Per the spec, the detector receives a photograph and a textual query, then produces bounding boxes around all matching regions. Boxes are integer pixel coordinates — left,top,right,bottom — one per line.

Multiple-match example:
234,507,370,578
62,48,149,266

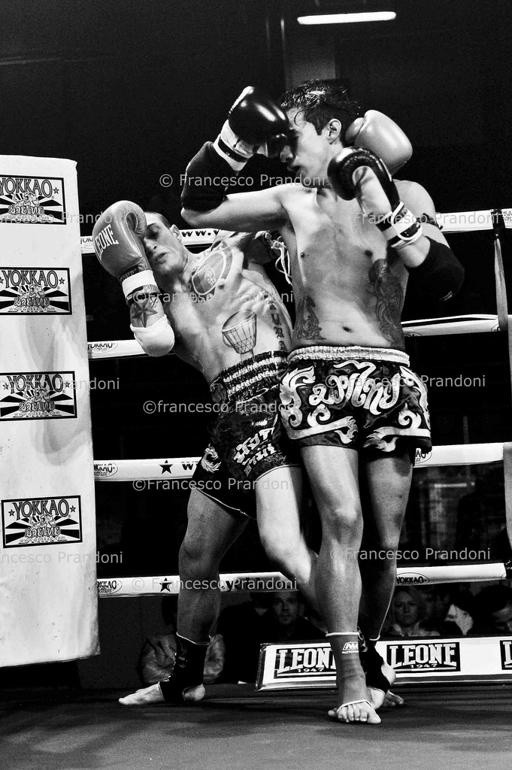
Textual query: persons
93,106,418,709
177,75,468,727
116,577,512,696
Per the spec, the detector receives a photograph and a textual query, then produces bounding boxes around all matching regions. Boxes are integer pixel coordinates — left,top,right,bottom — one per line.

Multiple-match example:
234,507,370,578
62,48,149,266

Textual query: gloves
89,197,162,305
209,84,291,172
325,145,422,251
342,106,417,179
